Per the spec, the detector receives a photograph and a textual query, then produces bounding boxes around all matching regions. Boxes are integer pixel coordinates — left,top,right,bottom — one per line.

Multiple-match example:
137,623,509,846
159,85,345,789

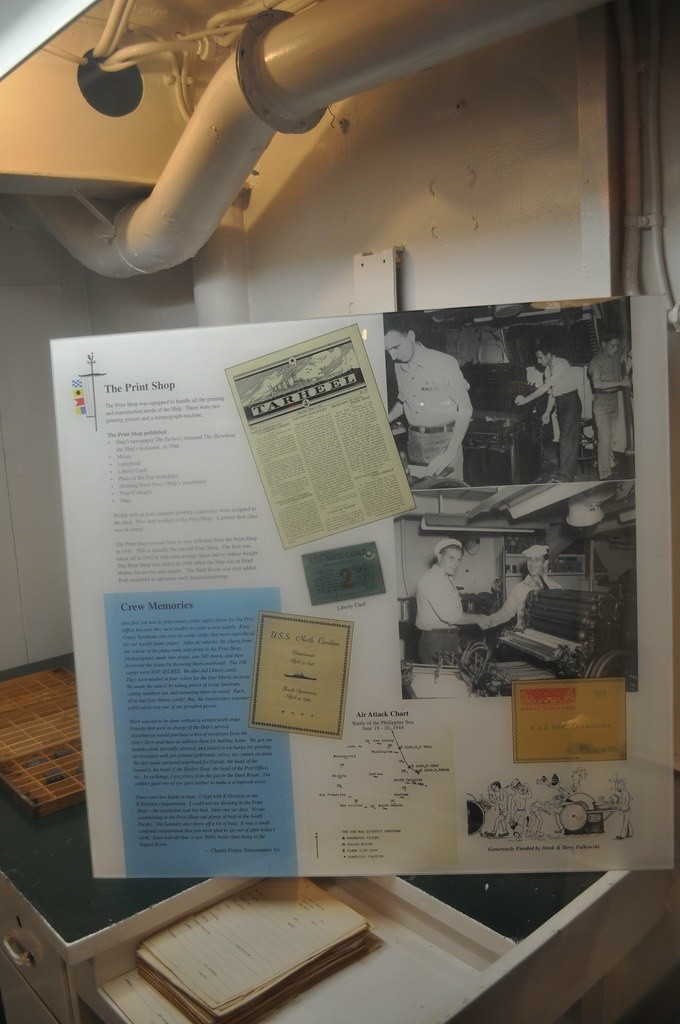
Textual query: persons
385,323,473,481
517,345,582,484
589,333,631,480
481,544,562,629
415,539,492,665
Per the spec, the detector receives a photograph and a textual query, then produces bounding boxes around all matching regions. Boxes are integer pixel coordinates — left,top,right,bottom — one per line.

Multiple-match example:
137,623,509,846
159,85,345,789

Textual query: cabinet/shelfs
0,652,680,1024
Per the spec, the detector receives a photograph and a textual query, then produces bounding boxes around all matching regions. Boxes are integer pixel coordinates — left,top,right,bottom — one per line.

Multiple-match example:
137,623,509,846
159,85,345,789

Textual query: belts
410,423,455,433
423,629,454,633
593,388,619,394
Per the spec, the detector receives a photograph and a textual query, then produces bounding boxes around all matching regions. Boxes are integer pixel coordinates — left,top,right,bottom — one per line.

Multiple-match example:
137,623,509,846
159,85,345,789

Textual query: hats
433,538,462,557
522,545,549,557
